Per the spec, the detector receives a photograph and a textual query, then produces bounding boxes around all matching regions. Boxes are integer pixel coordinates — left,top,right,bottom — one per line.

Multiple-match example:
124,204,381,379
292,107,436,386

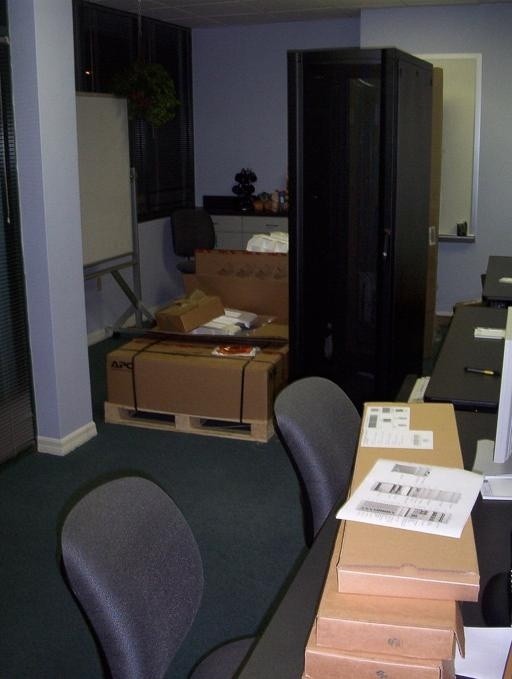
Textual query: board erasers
457,223,467,236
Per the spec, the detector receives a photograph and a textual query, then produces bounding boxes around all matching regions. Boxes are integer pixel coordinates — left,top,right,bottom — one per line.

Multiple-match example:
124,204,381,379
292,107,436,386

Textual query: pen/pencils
465,367,499,376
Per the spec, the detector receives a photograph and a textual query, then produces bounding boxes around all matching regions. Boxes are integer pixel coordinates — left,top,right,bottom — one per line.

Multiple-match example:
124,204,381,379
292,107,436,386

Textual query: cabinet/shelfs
290,48,434,403
205,215,288,255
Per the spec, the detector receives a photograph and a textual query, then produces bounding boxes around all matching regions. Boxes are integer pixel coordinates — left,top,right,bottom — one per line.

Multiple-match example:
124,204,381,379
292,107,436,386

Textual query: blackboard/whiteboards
76,92,135,269
412,53,482,243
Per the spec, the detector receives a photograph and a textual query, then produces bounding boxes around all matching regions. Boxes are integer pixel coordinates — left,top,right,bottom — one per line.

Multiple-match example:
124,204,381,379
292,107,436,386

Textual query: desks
481,255,511,307
422,303,511,410
233,407,511,678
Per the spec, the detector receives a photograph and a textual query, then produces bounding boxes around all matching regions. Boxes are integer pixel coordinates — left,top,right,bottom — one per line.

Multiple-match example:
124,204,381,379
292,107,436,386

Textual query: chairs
59,475,263,677
272,376,363,539
171,206,216,273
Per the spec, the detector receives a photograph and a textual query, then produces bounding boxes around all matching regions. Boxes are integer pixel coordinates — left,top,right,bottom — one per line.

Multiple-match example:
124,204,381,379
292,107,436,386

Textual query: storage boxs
104,247,290,420
